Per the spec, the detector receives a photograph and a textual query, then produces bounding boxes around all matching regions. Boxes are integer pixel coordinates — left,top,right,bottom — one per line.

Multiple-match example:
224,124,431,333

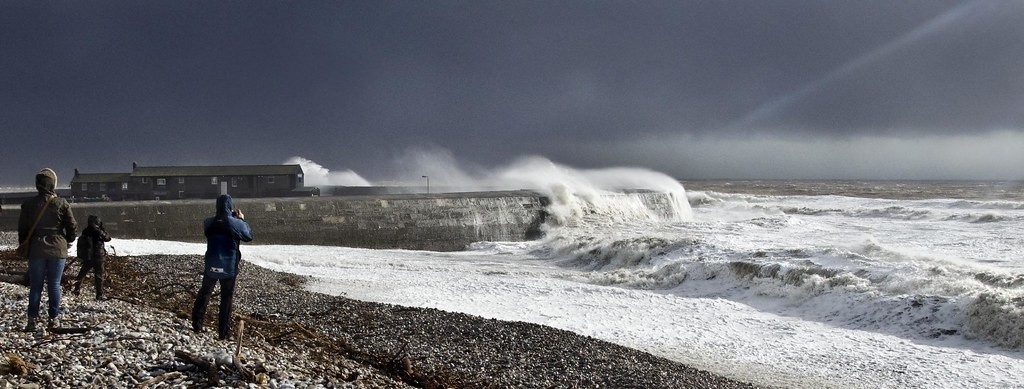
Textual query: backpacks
77,235,93,261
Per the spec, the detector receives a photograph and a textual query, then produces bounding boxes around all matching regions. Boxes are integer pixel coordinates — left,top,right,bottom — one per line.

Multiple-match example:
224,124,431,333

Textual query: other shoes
48,319,61,329
96,293,108,300
193,323,207,333
26,319,38,330
73,289,81,296
219,330,232,341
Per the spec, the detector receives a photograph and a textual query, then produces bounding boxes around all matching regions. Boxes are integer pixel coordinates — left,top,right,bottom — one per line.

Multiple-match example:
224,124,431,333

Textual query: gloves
99,222,105,232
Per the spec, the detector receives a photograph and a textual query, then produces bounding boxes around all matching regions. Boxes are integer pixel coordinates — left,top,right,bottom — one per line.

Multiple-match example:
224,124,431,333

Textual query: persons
74,215,111,299
18,168,78,330
192,194,253,339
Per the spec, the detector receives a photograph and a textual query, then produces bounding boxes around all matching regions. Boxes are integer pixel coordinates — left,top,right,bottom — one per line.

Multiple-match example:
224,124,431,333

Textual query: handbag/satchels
16,241,29,257
205,251,236,281
